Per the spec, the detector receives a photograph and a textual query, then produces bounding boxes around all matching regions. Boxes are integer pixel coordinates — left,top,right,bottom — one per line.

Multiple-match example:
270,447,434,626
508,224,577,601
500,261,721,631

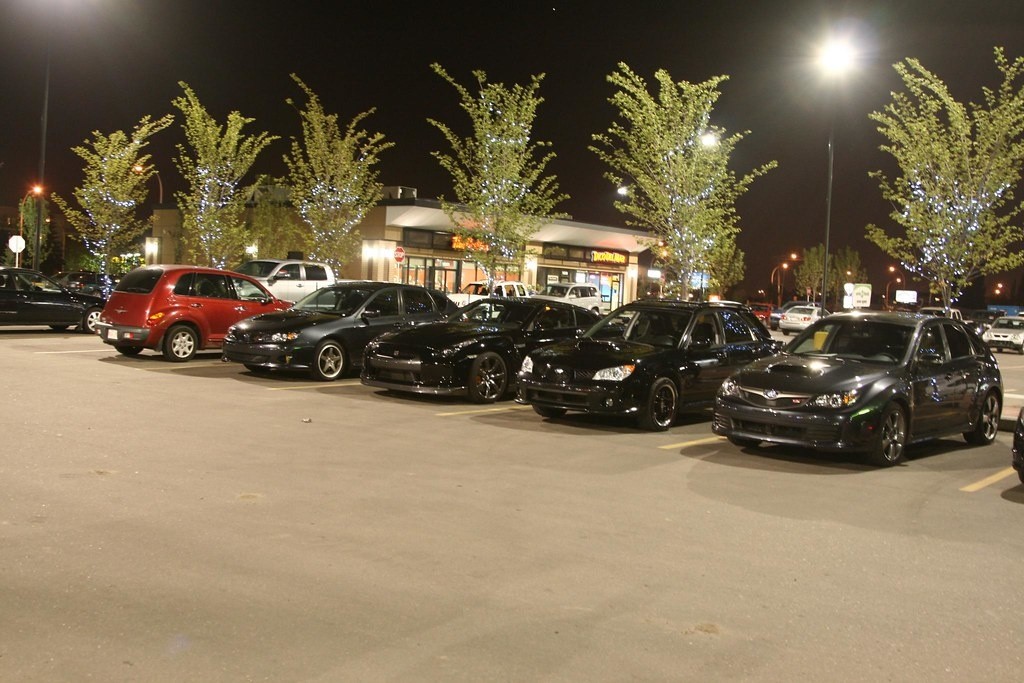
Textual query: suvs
917,306,1007,339
770,301,821,331
531,282,601,317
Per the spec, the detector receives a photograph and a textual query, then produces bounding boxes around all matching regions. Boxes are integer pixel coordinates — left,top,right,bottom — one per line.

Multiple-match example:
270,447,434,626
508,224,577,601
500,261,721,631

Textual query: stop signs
394,246,406,263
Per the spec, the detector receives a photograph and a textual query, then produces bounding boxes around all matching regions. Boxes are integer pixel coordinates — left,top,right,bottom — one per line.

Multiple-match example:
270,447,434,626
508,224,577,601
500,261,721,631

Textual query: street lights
819,39,854,320
135,165,163,204
15,186,43,280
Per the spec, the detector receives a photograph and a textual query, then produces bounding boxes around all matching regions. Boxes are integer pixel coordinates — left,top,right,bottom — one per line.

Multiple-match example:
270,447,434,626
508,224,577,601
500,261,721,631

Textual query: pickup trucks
446,280,532,319
232,259,389,307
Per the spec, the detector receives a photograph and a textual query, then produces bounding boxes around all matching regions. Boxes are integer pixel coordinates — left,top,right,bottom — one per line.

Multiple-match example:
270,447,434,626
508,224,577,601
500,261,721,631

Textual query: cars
711,308,1003,468
515,299,788,433
750,302,777,329
95,264,295,363
221,279,468,383
41,271,111,300
0,265,107,334
778,306,831,335
360,295,631,404
982,316,1024,355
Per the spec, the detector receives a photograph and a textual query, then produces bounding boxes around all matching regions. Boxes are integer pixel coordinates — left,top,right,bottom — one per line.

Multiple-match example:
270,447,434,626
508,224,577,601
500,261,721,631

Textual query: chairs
691,322,716,345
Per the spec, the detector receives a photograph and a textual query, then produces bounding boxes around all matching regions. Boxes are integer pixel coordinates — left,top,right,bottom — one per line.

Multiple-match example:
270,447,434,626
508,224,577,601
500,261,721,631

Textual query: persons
552,288,579,296
